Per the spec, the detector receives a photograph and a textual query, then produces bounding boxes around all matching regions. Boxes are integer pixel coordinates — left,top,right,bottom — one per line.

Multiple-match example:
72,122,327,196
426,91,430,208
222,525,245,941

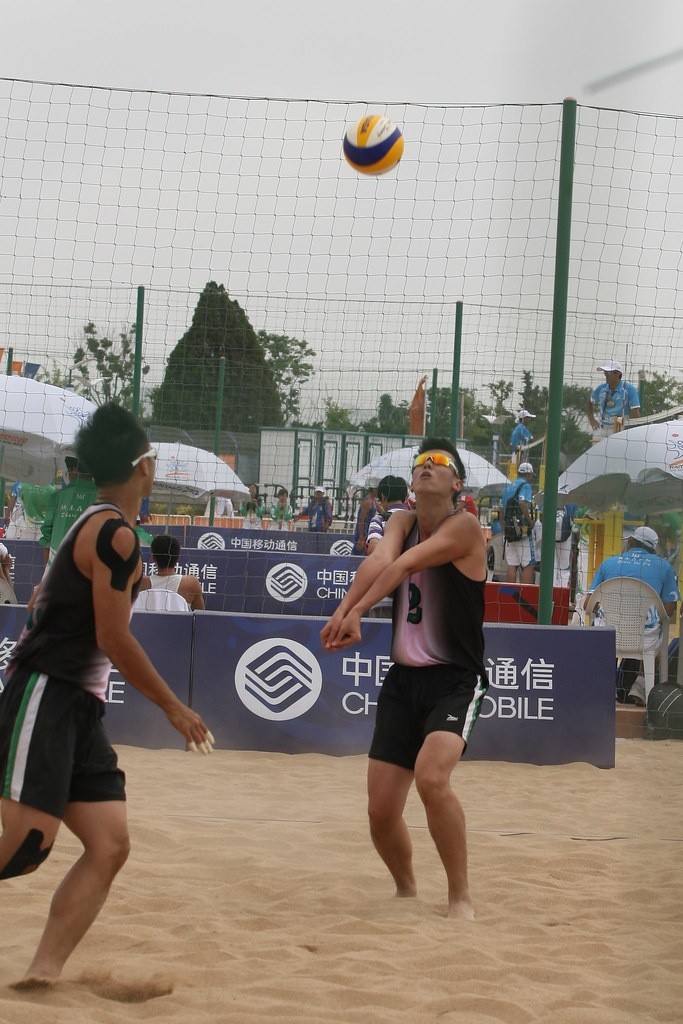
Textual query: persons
366,475,410,556
457,471,579,608
586,360,640,445
268,489,293,532
509,410,536,468
239,484,265,530
0,401,215,981
349,446,511,499
0,456,152,589
351,477,385,556
500,463,535,584
585,526,680,704
294,486,333,532
132,535,206,612
321,435,490,920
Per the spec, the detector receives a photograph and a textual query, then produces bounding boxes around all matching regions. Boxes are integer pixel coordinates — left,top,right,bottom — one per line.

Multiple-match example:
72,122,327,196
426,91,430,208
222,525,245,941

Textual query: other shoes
628,676,645,707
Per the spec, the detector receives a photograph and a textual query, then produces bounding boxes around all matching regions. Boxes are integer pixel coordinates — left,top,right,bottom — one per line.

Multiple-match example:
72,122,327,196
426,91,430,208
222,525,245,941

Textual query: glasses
412,453,460,479
131,448,157,467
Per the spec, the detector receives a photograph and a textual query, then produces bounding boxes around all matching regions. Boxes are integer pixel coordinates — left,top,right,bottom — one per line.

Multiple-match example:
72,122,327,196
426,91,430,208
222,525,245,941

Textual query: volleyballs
342,114,406,176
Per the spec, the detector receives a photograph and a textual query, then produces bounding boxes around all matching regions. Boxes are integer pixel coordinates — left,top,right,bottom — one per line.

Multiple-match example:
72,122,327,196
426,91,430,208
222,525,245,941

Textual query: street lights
49,358,98,392
70,374,112,401
482,414,513,466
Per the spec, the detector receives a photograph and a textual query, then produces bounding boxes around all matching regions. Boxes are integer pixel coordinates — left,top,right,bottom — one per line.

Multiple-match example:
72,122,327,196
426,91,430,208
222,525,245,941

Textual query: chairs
131,589,191,611
580,576,670,708
0,578,18,604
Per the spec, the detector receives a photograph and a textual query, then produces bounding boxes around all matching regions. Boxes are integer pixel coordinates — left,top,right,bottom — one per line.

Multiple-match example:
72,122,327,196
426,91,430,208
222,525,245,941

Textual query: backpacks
504,482,532,542
641,680,682,739
554,504,575,543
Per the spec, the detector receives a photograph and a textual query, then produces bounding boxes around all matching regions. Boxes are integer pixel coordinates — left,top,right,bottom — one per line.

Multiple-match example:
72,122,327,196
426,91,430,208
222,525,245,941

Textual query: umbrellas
558,421,683,495
0,374,100,487
149,440,251,535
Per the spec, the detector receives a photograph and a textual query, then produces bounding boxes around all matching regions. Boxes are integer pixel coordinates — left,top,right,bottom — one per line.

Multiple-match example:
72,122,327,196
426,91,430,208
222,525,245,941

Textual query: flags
409,376,429,435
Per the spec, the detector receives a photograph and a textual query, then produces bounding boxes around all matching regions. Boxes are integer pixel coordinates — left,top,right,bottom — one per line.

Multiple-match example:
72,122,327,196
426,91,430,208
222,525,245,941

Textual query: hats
315,486,325,492
597,361,623,378
518,410,536,418
621,527,659,550
365,477,382,488
518,462,536,476
